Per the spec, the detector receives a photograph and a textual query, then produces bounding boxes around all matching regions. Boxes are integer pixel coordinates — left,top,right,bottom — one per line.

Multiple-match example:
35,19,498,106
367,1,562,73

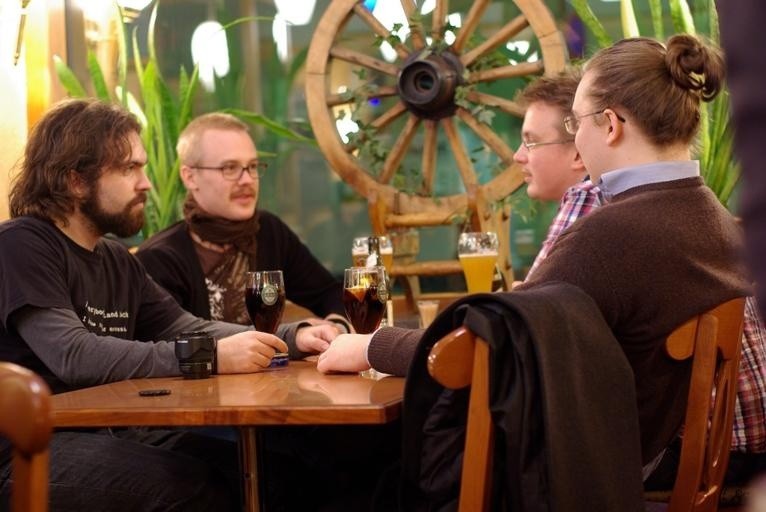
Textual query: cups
244,270,289,368
174,331,216,378
457,230,499,295
342,266,386,373
417,299,441,329
351,234,393,274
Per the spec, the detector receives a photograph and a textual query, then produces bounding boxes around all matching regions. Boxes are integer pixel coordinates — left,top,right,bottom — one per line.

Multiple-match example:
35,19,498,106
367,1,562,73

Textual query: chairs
641,297,746,512
428,328,493,511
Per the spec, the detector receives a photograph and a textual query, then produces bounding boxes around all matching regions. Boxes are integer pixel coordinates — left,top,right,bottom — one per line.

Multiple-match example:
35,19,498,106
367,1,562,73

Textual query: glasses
521,137,574,151
563,108,625,135
191,160,266,181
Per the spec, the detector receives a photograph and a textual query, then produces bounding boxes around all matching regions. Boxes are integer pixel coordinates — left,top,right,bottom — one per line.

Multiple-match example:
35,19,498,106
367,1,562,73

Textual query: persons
135,112,354,335
0,98,341,512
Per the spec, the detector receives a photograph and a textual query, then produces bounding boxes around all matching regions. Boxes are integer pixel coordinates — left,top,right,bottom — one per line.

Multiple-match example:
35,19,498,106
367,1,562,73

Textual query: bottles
364,236,393,327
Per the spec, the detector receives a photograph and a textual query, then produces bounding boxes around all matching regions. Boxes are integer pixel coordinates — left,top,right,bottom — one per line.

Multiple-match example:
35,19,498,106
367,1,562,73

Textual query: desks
42,360,427,512
